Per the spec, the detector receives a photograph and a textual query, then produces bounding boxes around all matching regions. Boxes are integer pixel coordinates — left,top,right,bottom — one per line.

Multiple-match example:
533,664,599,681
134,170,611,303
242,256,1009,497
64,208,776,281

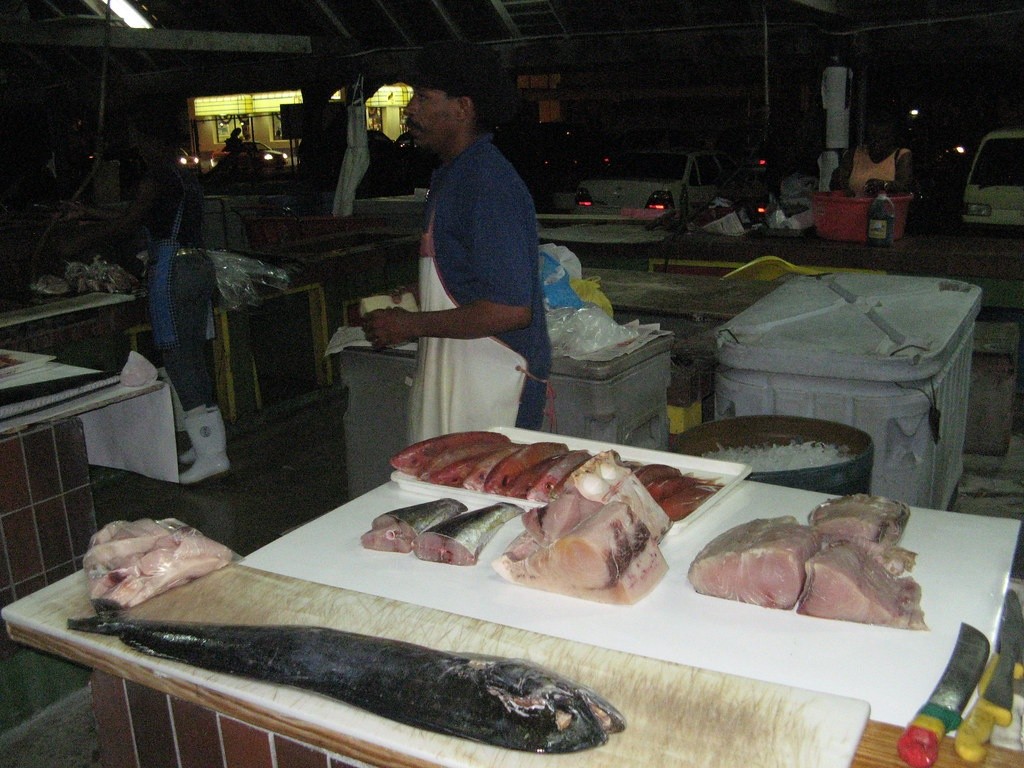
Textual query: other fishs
811,494,918,575
797,538,928,631
494,448,672,607
360,496,527,567
63,616,627,753
389,429,725,522
684,515,821,610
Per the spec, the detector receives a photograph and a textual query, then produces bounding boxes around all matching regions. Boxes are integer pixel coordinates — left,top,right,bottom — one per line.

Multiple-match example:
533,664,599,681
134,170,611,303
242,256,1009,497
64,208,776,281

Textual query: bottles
865,189,896,248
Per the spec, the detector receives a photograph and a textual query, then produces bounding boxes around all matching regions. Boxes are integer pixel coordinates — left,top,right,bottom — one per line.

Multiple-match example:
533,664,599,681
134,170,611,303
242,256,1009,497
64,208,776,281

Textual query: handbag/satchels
894,147,927,231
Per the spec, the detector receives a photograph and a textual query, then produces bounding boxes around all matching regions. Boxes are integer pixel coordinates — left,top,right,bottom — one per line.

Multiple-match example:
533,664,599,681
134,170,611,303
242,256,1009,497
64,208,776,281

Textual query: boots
180,404,206,464
179,408,231,485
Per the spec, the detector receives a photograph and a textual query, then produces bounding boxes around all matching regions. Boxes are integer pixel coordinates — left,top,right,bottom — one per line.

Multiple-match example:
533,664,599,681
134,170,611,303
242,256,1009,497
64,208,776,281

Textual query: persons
54,158,231,485
838,110,913,196
225,128,242,153
360,40,550,445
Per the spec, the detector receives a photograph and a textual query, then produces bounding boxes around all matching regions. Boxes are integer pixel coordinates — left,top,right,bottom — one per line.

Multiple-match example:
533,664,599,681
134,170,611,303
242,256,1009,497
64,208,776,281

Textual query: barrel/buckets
668,413,875,500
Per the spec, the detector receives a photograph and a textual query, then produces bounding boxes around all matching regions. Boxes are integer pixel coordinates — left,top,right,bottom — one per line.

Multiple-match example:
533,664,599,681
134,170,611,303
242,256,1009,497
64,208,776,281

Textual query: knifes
894,588,1024,768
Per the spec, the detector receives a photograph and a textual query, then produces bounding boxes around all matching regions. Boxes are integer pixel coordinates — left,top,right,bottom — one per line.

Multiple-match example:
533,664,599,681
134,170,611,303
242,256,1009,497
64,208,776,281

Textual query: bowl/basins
809,189,914,241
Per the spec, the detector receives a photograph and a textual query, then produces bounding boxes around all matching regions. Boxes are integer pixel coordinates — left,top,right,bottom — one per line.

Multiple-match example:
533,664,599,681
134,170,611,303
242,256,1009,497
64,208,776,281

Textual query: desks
229,227,421,297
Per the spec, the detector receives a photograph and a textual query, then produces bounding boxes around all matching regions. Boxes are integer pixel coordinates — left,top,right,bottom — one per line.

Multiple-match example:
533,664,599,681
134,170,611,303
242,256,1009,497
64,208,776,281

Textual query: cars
959,124,1024,227
173,145,200,172
570,145,738,217
210,140,289,170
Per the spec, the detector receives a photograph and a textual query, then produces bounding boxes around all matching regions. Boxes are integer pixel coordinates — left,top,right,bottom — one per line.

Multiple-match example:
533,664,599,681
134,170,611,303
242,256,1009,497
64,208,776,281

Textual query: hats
404,40,495,92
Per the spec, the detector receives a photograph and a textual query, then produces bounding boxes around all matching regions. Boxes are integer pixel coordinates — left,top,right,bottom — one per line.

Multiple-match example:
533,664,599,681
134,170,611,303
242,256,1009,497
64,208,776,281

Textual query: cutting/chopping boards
4,563,871,767
